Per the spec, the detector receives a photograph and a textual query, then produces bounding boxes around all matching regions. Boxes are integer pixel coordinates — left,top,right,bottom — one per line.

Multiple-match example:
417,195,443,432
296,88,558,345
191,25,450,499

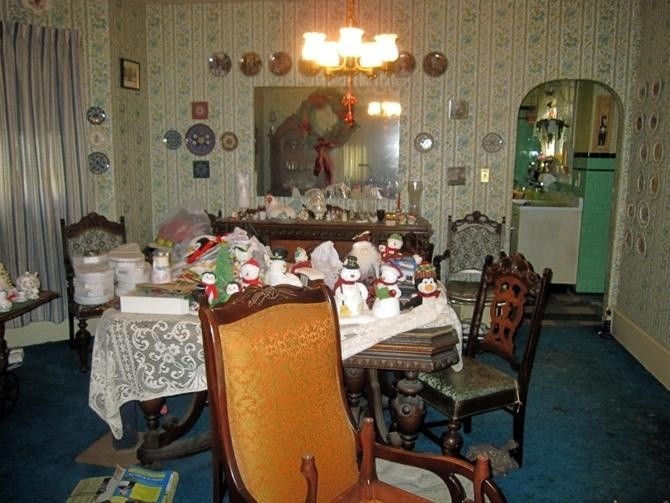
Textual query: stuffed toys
198,225,441,319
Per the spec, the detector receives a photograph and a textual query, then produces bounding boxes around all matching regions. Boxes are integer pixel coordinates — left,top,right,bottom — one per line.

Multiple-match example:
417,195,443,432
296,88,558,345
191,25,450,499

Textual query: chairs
417,252,553,465
60,211,127,351
197,278,504,502
432,210,507,322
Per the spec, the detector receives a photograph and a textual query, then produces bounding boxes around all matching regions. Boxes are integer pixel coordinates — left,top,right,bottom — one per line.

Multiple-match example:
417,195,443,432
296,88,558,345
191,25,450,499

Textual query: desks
0,290,61,417
102,264,460,464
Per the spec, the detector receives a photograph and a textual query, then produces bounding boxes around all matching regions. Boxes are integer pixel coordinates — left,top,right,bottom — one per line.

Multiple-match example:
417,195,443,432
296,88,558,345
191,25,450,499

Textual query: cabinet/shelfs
571,152,617,295
511,207,582,295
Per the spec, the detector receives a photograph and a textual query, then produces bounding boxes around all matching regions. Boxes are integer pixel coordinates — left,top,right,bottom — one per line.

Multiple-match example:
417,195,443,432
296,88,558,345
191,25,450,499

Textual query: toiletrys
525,183,533,199
533,187,542,200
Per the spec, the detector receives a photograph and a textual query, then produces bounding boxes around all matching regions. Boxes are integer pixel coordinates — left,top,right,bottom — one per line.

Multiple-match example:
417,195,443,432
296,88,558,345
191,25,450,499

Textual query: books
64,463,180,502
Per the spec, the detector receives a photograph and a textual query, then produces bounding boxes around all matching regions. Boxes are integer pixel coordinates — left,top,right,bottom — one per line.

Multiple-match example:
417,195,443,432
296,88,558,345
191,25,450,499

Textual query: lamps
298,1,402,130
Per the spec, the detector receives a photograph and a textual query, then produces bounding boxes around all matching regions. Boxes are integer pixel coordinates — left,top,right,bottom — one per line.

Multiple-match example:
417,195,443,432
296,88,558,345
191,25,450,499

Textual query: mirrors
251,84,404,200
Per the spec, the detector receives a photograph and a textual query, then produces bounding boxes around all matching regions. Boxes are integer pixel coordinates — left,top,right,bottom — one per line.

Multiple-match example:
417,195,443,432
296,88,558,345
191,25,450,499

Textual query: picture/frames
119,56,142,92
592,94,612,152
